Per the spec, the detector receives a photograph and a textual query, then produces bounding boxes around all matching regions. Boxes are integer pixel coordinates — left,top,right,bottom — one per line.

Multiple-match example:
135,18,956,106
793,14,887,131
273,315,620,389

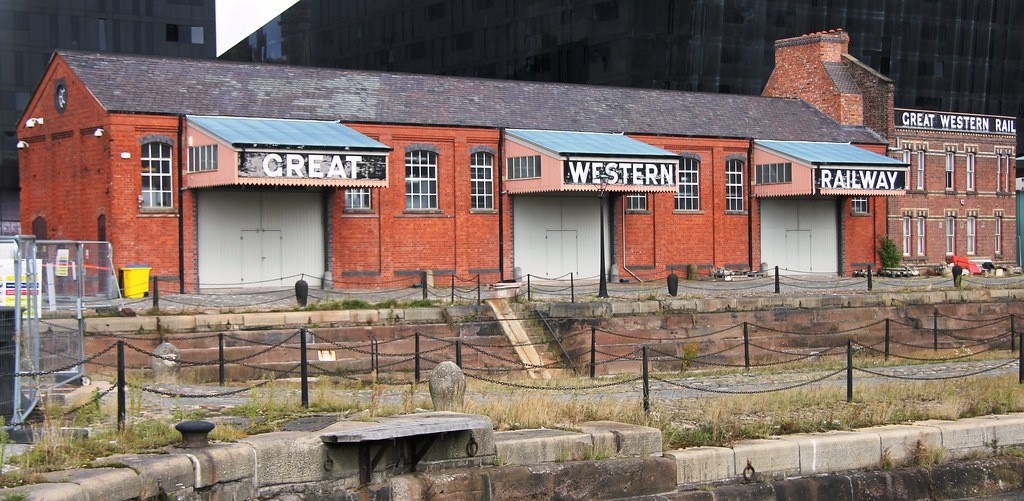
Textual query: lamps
16,140,29,149
25,117,44,129
94,128,104,138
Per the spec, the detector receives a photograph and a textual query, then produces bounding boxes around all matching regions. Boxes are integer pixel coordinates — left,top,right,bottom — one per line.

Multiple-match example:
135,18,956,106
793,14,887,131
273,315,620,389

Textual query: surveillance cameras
95,130,103,138
17,143,25,149
25,119,35,128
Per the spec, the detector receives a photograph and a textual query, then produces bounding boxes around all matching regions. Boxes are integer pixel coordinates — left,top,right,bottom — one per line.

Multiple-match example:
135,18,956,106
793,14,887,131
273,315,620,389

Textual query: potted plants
874,234,912,278
491,278,521,299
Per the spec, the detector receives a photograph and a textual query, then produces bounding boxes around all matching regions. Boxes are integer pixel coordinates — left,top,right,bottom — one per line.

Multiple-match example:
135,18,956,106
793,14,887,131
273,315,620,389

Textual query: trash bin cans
120,267,153,299
